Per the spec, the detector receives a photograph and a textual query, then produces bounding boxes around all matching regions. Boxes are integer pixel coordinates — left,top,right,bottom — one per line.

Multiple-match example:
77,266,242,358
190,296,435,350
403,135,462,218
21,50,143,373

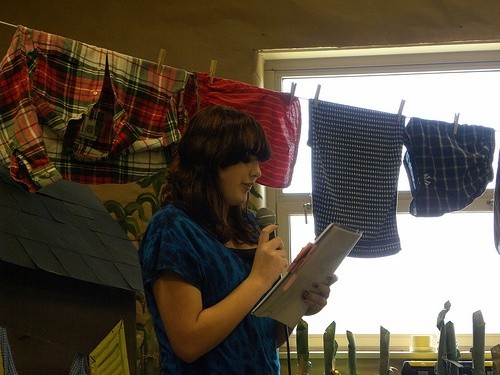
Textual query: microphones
256,208,282,283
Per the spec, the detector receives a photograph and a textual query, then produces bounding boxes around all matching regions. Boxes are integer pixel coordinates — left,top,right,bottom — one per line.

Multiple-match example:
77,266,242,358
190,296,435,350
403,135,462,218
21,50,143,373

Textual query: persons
137,106,338,375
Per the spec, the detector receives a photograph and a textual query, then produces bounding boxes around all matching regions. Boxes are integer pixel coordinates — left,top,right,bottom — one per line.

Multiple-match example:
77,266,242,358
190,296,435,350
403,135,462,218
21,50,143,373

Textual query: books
251,222,365,331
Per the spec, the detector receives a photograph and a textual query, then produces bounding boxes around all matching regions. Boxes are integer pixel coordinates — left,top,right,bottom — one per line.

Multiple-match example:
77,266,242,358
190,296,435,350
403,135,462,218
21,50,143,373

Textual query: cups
491,345,500,375
